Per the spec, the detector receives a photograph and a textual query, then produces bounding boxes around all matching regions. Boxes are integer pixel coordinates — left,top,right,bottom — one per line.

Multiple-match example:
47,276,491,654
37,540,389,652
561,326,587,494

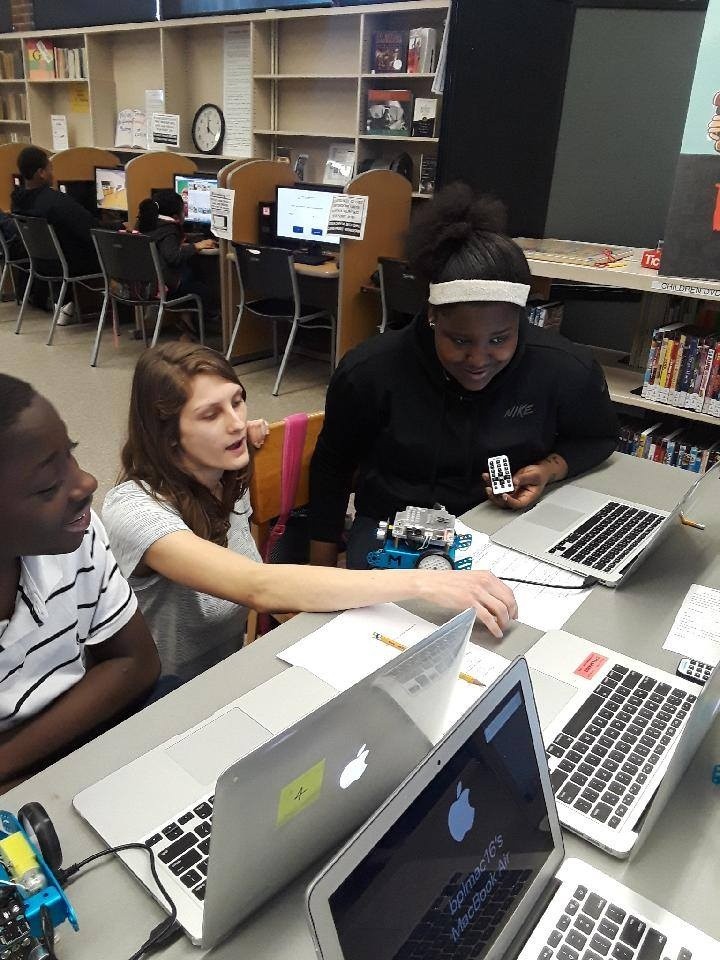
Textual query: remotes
488,455,515,496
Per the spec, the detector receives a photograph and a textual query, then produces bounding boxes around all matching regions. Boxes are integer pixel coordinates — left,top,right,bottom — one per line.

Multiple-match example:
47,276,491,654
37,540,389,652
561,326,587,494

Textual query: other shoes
56,307,87,325
175,318,198,339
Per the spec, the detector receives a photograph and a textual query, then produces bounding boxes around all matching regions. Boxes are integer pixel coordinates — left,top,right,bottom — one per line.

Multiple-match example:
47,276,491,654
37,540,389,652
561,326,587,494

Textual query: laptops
522,629,720,864
303,655,720,960
72,605,478,952
490,459,720,593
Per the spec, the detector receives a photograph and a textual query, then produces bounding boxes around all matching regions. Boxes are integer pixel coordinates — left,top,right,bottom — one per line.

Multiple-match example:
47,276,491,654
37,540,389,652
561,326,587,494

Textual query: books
0,48,30,144
525,296,562,332
418,153,438,195
26,38,86,79
322,141,356,187
411,96,441,140
365,87,414,137
614,318,720,477
368,26,410,74
406,26,438,75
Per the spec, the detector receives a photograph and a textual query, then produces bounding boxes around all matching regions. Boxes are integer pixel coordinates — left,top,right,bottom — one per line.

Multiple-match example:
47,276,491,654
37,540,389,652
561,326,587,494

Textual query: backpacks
110,224,184,299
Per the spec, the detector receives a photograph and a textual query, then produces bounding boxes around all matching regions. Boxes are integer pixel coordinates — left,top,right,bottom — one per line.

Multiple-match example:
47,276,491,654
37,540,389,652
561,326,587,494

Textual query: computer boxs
57,180,103,221
260,201,302,253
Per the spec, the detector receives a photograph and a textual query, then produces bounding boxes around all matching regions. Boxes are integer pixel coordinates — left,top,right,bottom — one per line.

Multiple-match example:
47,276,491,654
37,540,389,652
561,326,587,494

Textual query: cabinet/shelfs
509,236,720,426
0,0,453,199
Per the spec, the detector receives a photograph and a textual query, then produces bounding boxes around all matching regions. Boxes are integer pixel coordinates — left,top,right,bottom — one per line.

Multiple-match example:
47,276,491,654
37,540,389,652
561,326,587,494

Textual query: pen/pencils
372,630,488,688
685,519,706,530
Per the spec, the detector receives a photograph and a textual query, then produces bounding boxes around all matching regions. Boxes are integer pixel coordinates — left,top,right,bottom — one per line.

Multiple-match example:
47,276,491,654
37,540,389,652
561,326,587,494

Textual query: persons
8,145,100,327
101,341,519,686
1,372,165,787
120,190,213,338
304,181,616,566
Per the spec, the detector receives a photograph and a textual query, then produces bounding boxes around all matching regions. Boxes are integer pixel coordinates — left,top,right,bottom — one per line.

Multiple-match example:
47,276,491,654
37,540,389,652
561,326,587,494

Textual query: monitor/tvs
173,174,218,233
274,186,343,250
93,167,128,217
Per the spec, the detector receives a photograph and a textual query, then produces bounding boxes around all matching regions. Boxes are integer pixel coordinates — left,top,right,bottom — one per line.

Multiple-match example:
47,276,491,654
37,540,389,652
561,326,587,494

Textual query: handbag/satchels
246,413,352,639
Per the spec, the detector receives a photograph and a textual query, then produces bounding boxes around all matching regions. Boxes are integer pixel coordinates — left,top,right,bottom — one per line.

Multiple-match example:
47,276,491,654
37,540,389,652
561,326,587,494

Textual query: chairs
0,211,413,648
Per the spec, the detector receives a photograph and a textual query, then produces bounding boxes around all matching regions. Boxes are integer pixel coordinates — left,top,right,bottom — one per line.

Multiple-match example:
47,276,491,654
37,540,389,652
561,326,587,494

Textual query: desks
0,450,720,960
0,141,412,370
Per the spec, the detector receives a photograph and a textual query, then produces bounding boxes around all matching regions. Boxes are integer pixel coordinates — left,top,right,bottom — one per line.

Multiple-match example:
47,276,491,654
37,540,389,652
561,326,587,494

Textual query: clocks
192,103,225,154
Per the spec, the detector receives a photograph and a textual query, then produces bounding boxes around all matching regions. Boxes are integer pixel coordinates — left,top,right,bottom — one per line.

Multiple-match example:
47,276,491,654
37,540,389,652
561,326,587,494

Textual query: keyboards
288,251,335,266
183,232,217,243
